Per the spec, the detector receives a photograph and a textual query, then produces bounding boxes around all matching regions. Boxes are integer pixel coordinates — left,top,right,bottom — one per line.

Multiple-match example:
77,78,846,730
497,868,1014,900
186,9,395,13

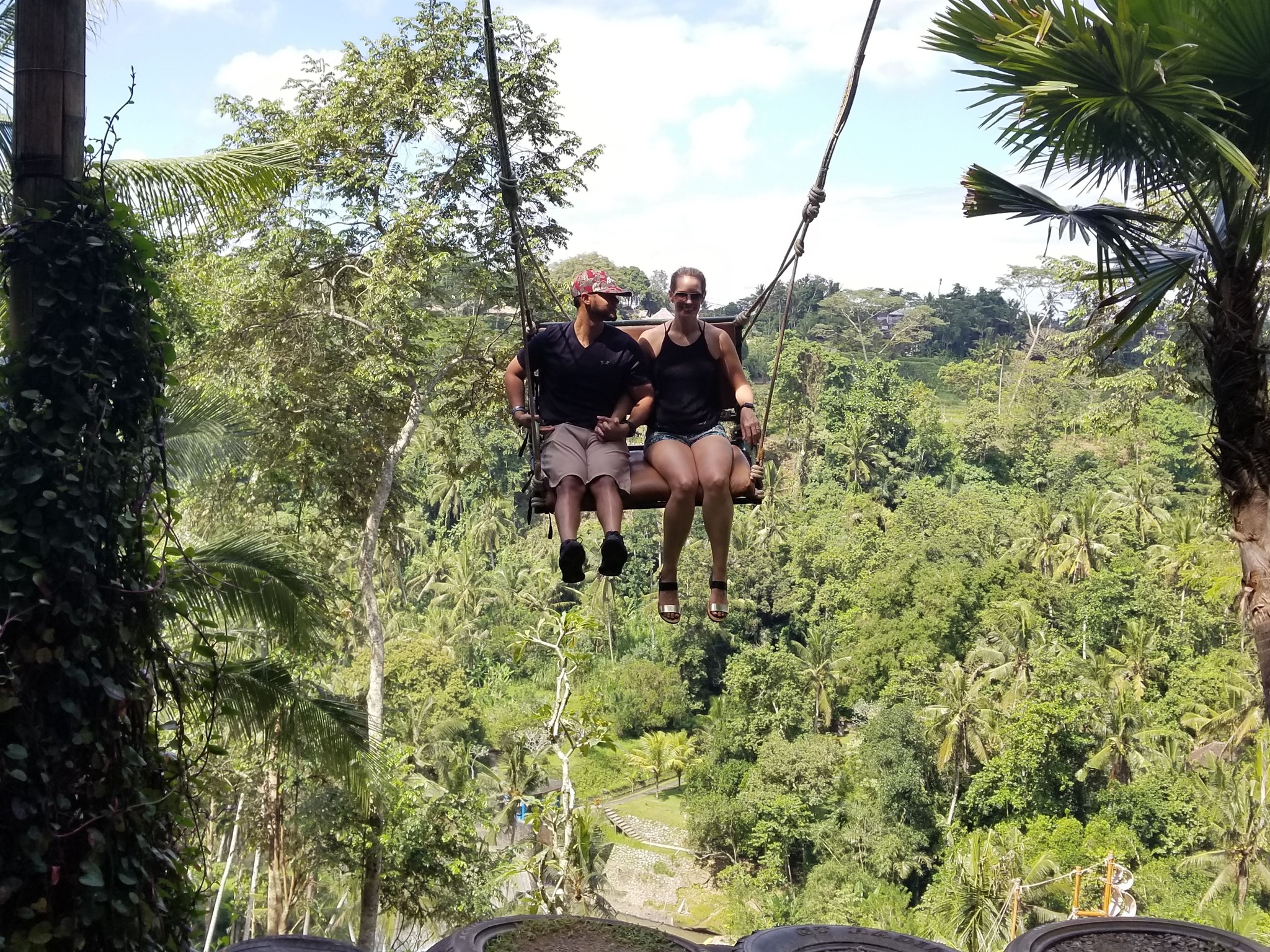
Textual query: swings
483,0,880,540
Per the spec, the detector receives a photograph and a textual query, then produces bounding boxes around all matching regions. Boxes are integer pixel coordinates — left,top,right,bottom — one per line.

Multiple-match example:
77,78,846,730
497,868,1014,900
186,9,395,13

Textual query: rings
756,426,760,430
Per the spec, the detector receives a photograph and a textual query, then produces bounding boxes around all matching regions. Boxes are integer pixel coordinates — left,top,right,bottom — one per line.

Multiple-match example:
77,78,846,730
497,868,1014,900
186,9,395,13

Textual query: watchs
741,402,754,412
623,420,635,437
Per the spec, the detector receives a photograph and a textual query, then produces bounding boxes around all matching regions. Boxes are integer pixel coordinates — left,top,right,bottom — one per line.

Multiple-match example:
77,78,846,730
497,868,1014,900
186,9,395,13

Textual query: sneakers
598,529,628,576
558,537,586,583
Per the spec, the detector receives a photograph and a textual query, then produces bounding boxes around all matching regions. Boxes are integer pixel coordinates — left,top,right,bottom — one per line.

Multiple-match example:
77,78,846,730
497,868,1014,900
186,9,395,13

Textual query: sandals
658,572,680,624
707,569,729,622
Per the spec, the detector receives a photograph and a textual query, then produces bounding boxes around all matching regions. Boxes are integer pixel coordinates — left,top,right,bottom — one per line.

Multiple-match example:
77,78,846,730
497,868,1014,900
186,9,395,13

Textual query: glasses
670,290,703,303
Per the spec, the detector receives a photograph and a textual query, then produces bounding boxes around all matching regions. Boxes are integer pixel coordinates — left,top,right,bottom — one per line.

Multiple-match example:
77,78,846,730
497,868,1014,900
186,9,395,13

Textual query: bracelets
511,406,526,417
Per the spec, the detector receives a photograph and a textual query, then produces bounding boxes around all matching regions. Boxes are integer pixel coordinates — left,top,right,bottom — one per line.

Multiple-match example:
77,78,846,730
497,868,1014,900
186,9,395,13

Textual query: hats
571,269,632,298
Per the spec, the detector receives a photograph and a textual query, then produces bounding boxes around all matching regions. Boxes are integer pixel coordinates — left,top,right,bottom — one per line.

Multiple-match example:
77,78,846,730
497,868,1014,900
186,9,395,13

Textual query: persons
596,267,761,624
505,268,653,583
